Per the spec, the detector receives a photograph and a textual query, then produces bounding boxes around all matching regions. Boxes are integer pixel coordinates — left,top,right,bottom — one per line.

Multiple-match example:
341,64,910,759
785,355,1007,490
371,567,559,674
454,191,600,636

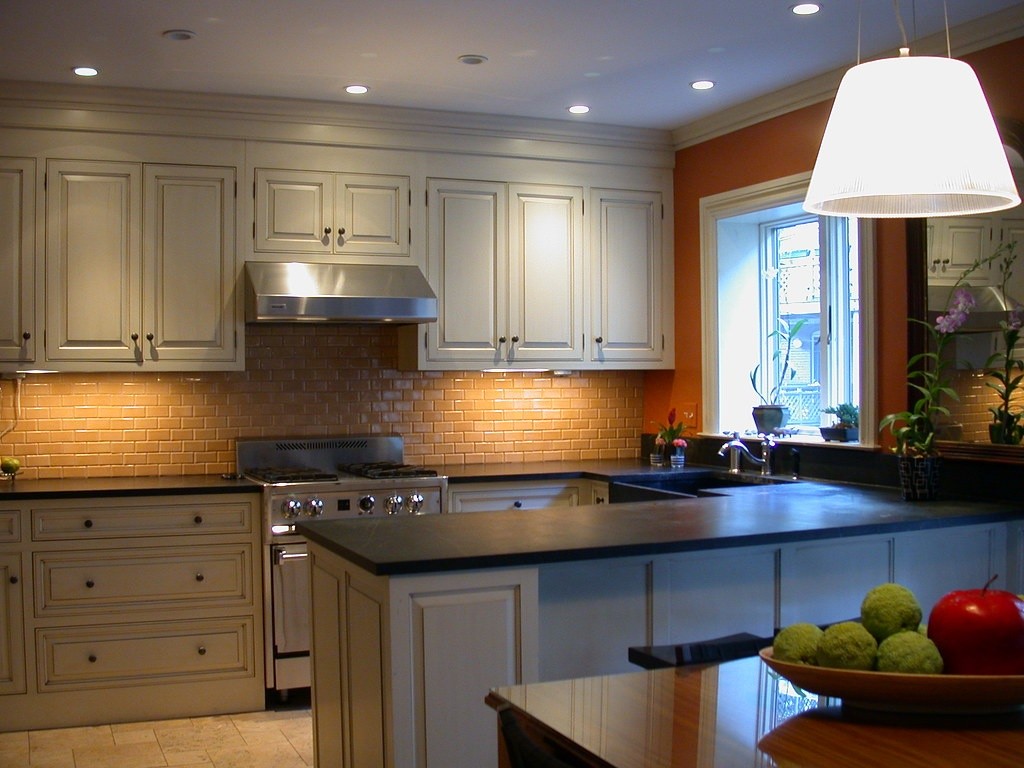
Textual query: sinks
607,472,804,498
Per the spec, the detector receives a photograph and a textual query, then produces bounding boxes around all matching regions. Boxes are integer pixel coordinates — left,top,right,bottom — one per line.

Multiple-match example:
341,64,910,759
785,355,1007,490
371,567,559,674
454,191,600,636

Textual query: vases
989,424,1023,445
751,404,792,438
898,451,937,498
649,445,685,468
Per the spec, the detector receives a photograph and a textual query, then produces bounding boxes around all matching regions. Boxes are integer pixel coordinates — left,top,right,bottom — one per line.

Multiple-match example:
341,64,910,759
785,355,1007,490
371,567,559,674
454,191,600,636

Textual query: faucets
717,432,777,476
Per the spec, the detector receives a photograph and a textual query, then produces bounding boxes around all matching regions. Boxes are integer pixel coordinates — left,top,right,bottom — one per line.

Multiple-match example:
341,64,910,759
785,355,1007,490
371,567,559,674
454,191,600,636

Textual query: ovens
263,476,449,710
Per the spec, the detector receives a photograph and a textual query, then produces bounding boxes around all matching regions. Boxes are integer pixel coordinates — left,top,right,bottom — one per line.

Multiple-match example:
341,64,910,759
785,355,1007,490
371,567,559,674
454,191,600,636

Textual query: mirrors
908,114,1024,451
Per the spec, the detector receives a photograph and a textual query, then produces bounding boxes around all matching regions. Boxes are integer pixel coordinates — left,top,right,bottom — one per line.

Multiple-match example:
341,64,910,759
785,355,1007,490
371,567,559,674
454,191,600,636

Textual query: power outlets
682,402,697,429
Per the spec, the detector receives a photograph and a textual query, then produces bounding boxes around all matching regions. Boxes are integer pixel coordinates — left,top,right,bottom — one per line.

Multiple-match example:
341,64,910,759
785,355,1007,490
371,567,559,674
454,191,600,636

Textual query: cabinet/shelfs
248,96,414,266
447,477,605,514
0,89,40,376
397,116,583,371
39,81,247,373
0,495,266,735
581,128,678,370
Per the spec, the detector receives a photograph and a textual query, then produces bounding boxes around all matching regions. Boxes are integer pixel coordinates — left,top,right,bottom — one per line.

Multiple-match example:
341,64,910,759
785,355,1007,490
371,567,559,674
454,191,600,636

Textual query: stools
627,615,862,670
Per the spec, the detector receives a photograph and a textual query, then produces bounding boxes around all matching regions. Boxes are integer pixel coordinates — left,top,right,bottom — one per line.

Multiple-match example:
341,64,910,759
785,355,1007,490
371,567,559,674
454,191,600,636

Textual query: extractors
244,260,437,323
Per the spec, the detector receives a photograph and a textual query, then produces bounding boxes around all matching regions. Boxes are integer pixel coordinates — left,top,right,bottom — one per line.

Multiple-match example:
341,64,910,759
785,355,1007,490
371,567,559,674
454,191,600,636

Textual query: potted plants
819,404,859,442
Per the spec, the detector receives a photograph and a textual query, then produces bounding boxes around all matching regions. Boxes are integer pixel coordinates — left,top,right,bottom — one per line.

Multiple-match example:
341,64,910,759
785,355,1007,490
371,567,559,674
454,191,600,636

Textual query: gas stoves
241,462,439,484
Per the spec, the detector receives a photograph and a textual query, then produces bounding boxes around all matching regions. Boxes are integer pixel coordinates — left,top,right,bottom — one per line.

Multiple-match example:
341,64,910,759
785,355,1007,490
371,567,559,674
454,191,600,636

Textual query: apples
927,573,1024,676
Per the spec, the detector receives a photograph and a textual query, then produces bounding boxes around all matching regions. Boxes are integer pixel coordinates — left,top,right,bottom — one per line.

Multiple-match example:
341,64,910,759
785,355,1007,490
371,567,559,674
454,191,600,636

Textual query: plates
758,647,1024,702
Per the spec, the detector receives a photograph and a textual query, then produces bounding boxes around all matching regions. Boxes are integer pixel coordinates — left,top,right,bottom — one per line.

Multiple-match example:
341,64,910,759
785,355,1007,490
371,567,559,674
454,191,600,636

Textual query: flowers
882,259,981,452
748,266,807,407
651,409,694,445
988,240,1024,424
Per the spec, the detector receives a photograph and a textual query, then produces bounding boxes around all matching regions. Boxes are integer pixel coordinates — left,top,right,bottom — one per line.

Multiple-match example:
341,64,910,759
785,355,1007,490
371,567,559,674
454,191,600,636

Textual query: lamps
803,0,1020,220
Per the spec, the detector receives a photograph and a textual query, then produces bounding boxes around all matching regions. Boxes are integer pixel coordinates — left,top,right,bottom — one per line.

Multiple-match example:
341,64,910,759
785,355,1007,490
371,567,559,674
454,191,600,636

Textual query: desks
484,654,1024,768
